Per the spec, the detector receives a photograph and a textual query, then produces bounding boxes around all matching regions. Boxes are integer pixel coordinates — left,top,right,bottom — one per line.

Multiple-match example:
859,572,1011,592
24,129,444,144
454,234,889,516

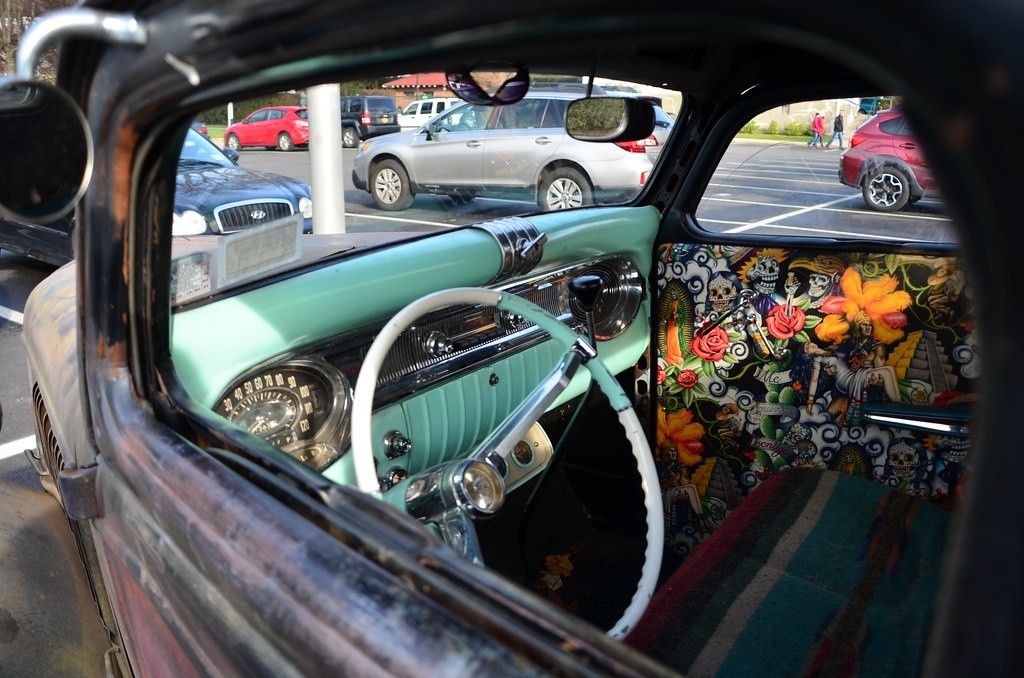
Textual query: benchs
600,468,952,678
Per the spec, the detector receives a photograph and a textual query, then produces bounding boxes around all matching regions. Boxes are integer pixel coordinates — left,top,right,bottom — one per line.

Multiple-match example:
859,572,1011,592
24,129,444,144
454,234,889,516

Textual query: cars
223,106,310,152
0,0,1024,678
0,125,313,267
190,120,212,140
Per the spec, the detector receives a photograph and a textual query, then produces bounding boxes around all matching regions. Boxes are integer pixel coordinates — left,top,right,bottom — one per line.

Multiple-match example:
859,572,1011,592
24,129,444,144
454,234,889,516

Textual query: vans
396,96,463,133
858,97,879,116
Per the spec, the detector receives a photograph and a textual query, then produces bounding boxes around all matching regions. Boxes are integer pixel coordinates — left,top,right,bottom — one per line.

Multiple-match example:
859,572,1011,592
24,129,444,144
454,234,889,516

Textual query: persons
826,110,844,150
807,113,827,149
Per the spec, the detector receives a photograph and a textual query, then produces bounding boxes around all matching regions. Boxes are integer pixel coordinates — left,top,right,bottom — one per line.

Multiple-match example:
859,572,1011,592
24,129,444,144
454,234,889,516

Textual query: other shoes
808,142,811,148
826,145,830,150
813,144,818,149
809,145,816,150
821,146,826,149
839,146,845,150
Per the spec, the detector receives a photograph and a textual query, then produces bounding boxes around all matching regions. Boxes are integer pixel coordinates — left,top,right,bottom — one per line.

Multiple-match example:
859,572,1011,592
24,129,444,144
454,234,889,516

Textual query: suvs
354,85,675,216
837,102,956,213
340,94,400,148
